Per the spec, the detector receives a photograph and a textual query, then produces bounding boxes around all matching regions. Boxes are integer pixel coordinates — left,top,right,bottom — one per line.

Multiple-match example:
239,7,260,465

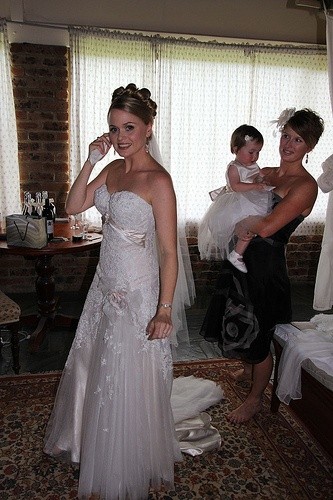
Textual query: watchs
158,303,172,310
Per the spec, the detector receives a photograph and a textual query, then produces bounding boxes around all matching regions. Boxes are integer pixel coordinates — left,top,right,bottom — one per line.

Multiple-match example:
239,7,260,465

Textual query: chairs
0,291,21,375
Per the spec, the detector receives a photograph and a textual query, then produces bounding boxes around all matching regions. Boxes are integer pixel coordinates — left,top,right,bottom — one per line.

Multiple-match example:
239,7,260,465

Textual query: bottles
53,218,71,239
22,191,56,241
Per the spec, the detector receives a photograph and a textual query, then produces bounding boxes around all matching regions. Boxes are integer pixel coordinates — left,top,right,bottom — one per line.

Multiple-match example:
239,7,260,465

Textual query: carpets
0,357,333,500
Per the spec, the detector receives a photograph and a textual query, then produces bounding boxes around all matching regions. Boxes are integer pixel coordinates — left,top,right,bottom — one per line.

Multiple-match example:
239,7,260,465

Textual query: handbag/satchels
5,212,47,249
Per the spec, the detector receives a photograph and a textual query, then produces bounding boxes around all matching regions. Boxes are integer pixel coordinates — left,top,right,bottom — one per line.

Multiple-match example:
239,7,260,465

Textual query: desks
0,233,103,355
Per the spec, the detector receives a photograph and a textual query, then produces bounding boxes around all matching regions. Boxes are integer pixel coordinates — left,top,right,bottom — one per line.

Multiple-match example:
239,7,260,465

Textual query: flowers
271,106,296,127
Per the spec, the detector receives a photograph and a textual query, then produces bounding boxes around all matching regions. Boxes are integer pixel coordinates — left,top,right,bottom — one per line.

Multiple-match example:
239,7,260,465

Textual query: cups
72,222,89,242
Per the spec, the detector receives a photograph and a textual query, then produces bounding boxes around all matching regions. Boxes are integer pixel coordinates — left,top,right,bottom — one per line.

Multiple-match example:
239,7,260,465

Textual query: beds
271,313,333,442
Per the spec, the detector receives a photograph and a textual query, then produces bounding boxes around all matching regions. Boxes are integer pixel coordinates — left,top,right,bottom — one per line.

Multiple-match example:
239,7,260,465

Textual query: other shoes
227,250,247,273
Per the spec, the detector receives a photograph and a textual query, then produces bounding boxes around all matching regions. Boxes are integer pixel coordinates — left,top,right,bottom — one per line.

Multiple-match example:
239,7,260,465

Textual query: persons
199,125,275,272
200,108,325,426
44,82,225,500
